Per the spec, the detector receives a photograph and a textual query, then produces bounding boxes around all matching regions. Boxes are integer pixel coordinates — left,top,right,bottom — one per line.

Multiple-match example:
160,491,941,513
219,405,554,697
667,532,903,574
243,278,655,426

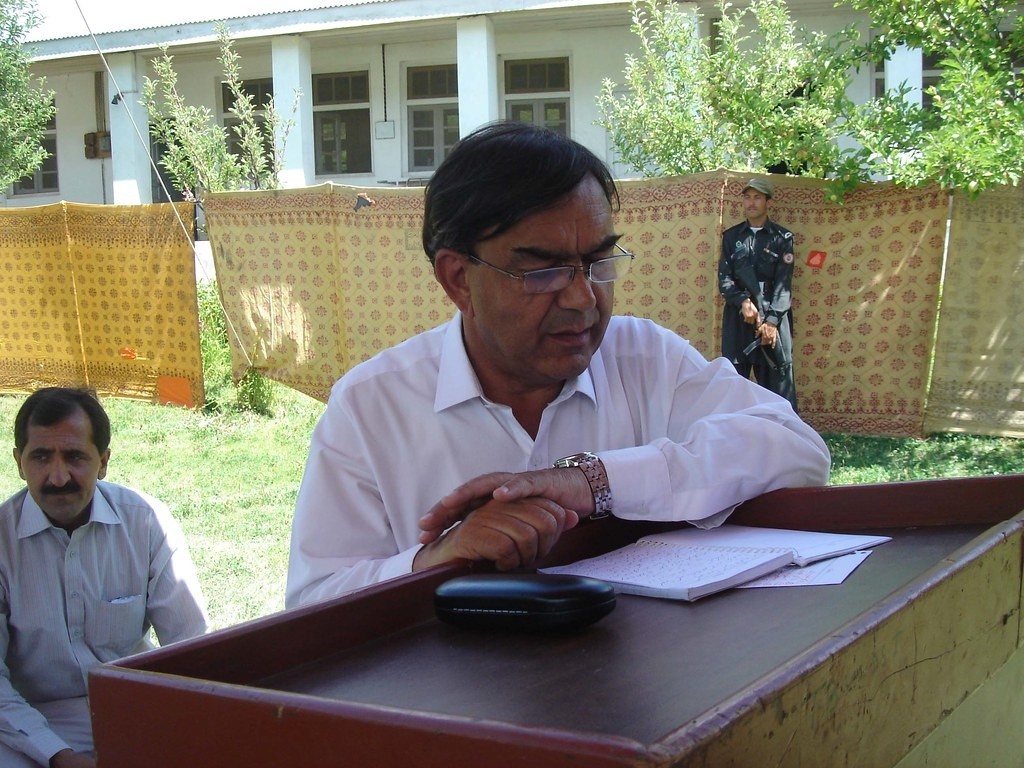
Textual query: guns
732,263,792,378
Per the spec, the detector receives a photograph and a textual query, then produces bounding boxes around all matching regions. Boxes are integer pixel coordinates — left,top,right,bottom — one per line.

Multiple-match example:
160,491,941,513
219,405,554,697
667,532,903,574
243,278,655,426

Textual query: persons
0,388,210,768
284,121,831,615
717,179,799,414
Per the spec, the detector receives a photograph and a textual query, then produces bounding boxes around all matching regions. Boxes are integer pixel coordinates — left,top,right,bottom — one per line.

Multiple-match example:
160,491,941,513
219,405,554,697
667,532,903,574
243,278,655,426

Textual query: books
537,523,892,603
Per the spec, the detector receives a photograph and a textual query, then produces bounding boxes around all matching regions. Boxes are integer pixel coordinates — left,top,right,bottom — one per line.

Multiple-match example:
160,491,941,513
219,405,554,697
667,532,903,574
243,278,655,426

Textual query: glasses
467,244,637,294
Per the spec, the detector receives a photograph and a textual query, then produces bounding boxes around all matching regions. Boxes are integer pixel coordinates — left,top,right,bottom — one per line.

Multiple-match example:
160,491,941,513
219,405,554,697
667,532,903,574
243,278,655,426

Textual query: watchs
552,451,613,521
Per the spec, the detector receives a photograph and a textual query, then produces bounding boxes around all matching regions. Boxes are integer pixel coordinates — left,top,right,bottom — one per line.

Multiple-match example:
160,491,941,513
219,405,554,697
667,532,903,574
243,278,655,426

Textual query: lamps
112,90,138,105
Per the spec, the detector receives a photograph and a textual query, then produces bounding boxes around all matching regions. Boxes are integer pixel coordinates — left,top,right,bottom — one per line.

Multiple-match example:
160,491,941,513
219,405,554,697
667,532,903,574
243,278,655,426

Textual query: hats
741,178,774,199
